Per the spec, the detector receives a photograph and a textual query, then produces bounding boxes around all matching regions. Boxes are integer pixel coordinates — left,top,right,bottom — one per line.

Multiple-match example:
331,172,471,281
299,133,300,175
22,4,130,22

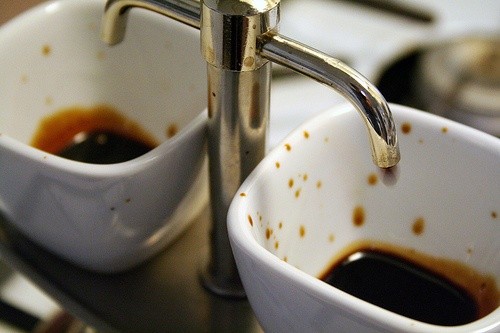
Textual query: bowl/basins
0,0,211,276
226,98,500,333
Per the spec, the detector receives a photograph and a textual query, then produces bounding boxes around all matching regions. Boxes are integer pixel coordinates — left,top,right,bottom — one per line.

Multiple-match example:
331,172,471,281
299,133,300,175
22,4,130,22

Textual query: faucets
102,0,402,299
